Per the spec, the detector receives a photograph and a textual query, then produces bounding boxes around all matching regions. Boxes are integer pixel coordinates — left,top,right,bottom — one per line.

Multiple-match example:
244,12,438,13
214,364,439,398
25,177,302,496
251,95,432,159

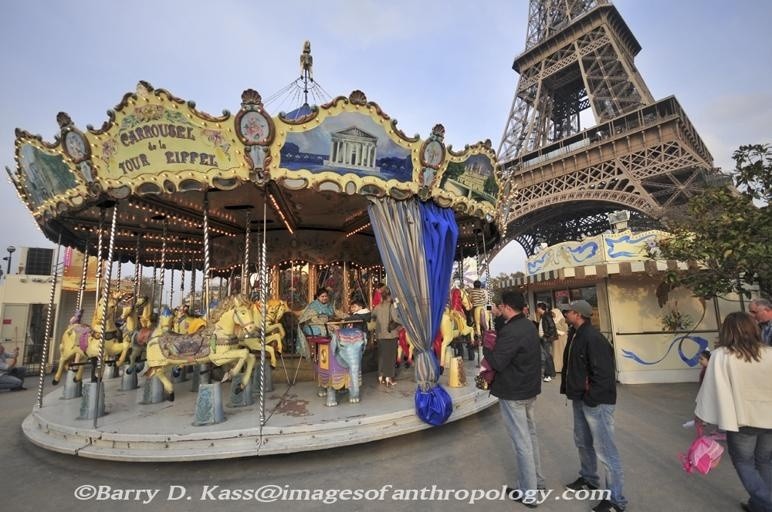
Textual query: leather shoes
506,487,536,508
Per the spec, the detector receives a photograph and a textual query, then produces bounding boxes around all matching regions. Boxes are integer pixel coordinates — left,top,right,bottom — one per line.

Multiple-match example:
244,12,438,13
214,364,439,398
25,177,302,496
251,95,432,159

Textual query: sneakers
591,499,624,512
740,502,750,512
566,477,598,491
542,375,554,381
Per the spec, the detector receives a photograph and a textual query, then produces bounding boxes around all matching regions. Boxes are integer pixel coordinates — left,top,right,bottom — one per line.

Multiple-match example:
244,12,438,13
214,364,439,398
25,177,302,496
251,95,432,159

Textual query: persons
301,287,336,336
484,289,547,507
748,298,772,346
342,283,404,386
444,280,559,381
0,343,28,391
559,300,628,512
694,312,772,512
696,349,727,440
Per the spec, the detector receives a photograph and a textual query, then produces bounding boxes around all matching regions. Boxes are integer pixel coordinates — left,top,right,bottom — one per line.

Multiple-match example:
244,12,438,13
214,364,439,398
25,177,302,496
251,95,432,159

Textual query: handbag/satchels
687,435,724,475
389,321,400,330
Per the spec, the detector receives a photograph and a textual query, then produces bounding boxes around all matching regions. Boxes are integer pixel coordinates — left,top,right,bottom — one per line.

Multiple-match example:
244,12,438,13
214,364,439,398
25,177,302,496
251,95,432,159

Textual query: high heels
386,377,397,387
378,376,385,385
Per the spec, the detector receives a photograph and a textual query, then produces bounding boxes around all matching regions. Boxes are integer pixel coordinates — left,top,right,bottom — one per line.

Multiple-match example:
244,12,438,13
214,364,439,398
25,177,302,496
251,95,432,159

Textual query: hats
560,300,592,319
